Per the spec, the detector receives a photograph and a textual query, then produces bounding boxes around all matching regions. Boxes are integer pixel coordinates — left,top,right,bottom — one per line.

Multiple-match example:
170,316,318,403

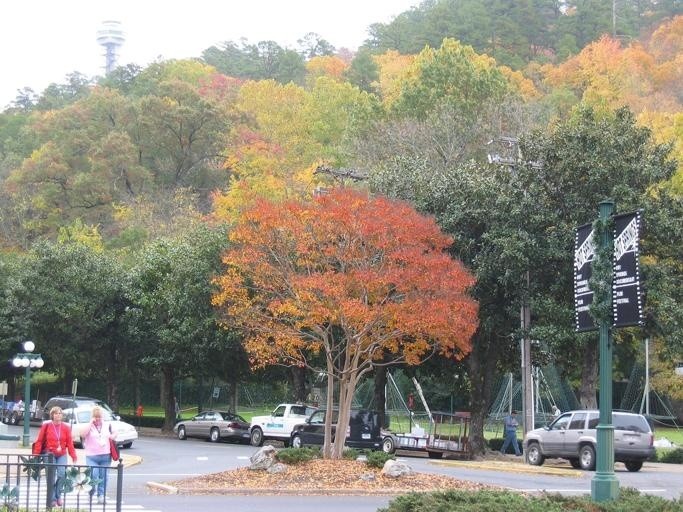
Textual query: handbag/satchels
32,420,48,455
110,441,119,461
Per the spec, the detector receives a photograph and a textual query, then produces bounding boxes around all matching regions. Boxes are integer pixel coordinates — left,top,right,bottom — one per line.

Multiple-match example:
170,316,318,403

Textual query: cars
42,396,138,449
173,404,382,450
522,409,656,472
3,402,14,416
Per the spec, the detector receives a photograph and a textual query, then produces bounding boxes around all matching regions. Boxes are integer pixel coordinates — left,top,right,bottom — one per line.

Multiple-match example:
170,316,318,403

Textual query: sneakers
52,498,63,507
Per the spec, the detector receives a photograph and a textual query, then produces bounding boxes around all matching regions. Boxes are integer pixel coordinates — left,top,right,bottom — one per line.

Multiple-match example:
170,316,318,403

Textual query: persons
552,406,561,416
500,410,522,456
136,401,144,426
32,406,78,507
78,404,120,504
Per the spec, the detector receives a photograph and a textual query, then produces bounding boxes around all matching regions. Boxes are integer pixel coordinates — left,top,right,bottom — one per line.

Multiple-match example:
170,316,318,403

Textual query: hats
511,410,518,414
552,406,557,412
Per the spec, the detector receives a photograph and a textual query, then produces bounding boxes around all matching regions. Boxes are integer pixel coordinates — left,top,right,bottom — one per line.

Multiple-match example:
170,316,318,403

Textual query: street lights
13,341,44,446
